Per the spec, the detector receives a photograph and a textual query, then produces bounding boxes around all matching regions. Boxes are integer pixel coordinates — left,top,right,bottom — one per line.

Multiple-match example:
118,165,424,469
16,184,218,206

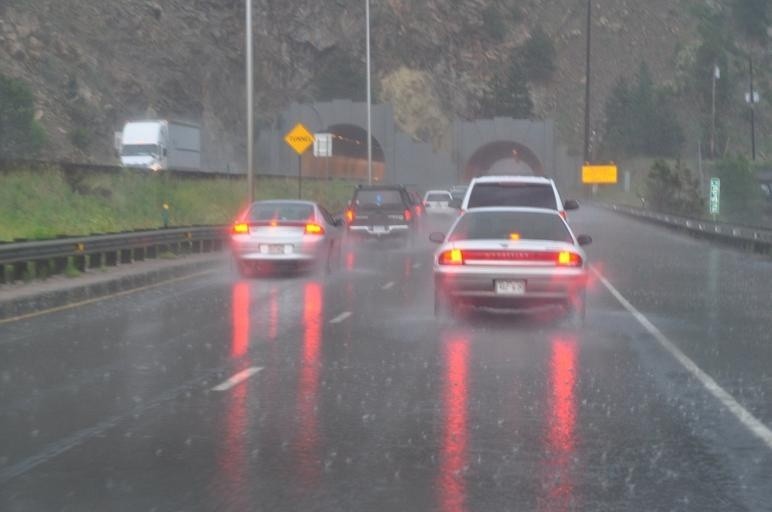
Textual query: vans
448,174,580,221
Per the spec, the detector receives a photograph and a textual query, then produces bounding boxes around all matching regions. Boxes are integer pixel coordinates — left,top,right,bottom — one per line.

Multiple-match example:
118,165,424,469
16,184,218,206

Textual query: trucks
120,118,202,171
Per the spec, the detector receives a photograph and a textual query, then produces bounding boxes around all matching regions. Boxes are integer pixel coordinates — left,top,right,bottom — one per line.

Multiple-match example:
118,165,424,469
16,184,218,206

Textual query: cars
345,184,468,241
429,207,592,323
230,200,345,276
